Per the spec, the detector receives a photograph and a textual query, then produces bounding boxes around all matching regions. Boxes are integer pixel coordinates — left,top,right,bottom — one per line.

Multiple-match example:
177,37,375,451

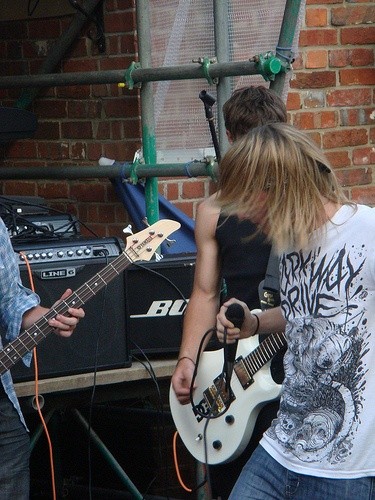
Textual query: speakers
0,255,132,384
128,255,223,354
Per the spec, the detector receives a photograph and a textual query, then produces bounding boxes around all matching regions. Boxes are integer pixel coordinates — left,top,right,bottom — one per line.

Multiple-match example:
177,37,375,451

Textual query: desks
13,358,213,500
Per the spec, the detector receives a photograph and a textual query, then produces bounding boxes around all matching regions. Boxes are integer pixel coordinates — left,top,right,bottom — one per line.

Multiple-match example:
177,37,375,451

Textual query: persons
206,122,375,500
171,86,288,500
0,215,85,500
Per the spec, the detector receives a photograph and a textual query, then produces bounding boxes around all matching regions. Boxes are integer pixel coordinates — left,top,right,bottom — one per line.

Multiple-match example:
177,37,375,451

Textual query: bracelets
175,356,196,367
252,312,260,337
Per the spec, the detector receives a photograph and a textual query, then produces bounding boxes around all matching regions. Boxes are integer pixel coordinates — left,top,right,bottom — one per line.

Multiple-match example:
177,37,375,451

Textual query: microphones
225,304,245,392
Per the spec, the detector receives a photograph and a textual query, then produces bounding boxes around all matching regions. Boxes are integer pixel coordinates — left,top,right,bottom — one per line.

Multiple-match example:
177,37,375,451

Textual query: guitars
0,217,182,377
168,309,289,465
